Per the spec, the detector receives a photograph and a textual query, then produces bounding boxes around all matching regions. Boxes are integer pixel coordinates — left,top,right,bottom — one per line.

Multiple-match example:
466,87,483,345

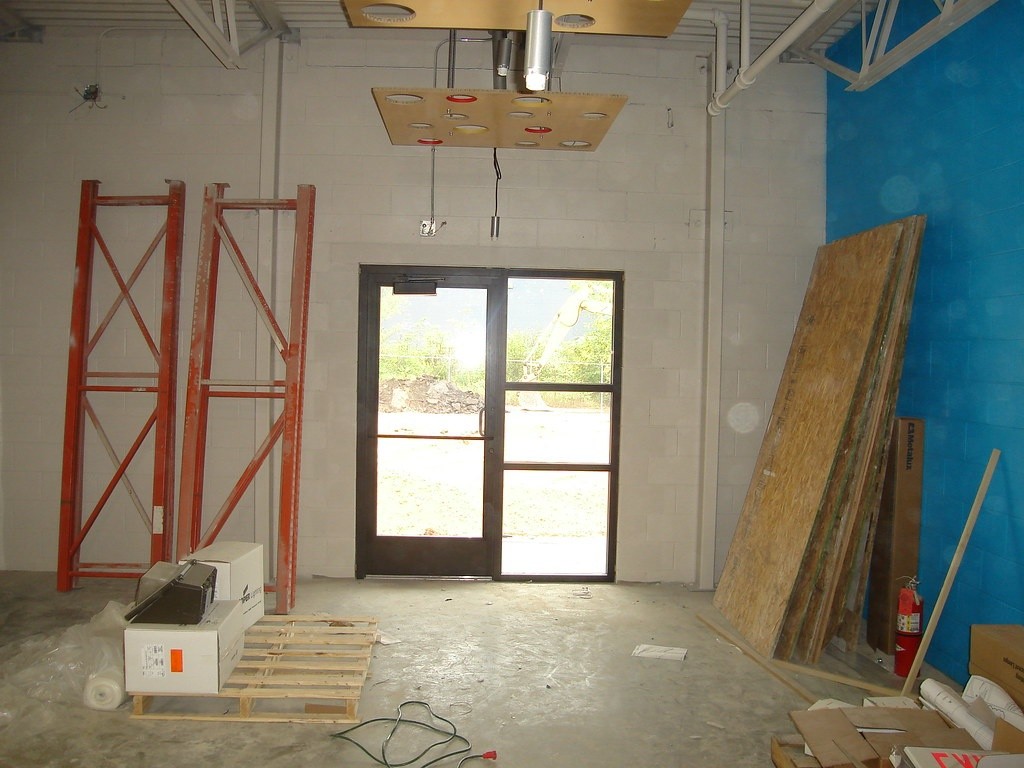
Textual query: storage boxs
866,418,924,652
967,624,1024,714
124,599,246,694
179,541,265,632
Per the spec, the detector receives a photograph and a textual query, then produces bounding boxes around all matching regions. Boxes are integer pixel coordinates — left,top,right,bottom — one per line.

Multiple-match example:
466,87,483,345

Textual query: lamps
523,0,555,90
496,30,513,77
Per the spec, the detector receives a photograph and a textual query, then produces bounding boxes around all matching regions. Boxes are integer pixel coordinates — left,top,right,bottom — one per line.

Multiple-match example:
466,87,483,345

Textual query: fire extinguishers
894,575,923,677
